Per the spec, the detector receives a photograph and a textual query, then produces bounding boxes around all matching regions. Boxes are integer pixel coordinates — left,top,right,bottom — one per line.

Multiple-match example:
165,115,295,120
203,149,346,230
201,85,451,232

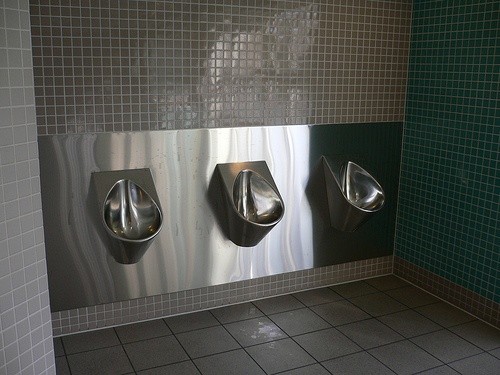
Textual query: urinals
85,167,165,265
321,147,388,232
206,159,286,248
